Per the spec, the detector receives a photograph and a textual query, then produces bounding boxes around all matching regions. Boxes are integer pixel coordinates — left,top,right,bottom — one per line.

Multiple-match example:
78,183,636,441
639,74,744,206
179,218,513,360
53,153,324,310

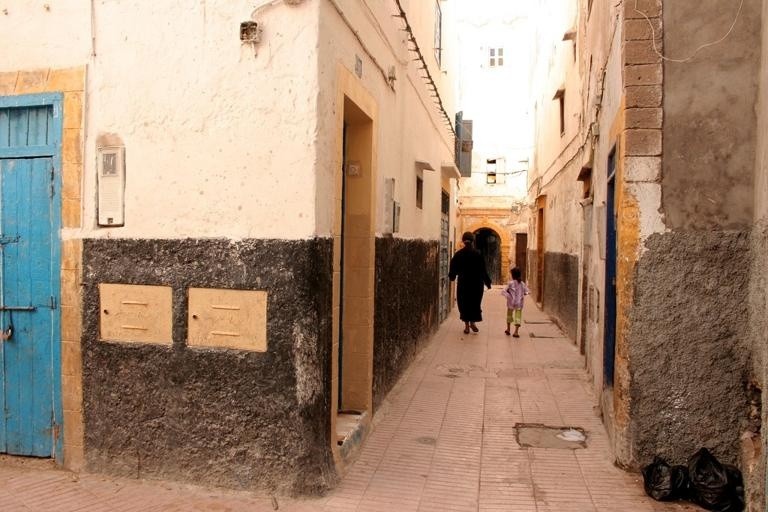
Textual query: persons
500,267,529,338
447,232,493,334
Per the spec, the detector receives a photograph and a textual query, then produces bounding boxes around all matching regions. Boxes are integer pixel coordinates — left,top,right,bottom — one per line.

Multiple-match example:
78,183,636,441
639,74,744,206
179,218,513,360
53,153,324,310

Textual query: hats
511,267,520,272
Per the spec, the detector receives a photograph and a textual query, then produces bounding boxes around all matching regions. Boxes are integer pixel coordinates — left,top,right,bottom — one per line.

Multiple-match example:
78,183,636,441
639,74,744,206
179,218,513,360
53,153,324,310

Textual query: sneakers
505,330,510,335
464,329,468,333
513,333,519,337
469,324,478,332
